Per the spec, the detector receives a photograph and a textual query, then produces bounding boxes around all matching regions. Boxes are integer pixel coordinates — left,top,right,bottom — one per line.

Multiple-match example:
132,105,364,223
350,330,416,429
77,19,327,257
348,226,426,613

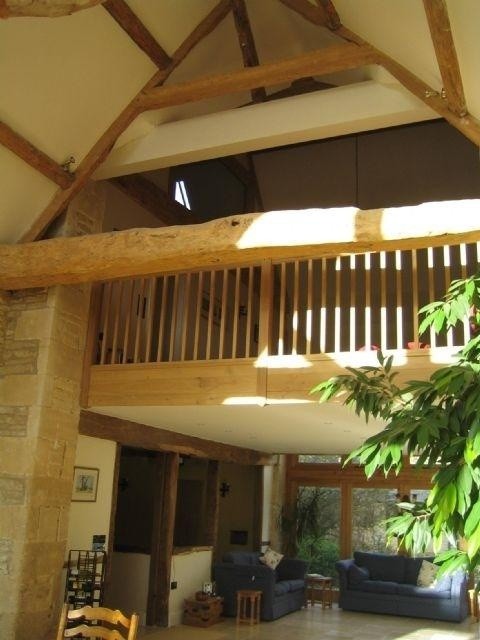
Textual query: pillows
353,552,442,587
258,546,284,571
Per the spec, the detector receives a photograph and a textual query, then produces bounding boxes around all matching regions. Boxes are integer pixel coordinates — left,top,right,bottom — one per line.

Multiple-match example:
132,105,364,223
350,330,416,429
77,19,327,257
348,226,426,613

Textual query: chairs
55,602,140,640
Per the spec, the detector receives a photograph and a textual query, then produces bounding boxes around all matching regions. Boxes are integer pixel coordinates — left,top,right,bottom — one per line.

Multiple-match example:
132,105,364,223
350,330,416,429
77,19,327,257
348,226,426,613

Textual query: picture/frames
198,288,231,333
70,466,100,502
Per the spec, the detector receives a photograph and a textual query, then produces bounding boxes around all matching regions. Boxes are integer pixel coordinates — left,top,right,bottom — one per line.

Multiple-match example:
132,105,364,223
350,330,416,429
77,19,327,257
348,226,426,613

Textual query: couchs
214,550,306,622
335,550,470,623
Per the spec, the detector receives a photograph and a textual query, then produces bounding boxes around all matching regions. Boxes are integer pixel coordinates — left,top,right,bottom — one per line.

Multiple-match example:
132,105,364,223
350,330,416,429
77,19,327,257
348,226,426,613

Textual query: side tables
236,588,262,627
301,573,334,610
183,596,225,628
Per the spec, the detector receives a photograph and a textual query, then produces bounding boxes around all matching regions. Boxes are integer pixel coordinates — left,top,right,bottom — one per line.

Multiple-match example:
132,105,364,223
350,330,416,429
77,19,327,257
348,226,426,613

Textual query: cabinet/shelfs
61,549,106,640
